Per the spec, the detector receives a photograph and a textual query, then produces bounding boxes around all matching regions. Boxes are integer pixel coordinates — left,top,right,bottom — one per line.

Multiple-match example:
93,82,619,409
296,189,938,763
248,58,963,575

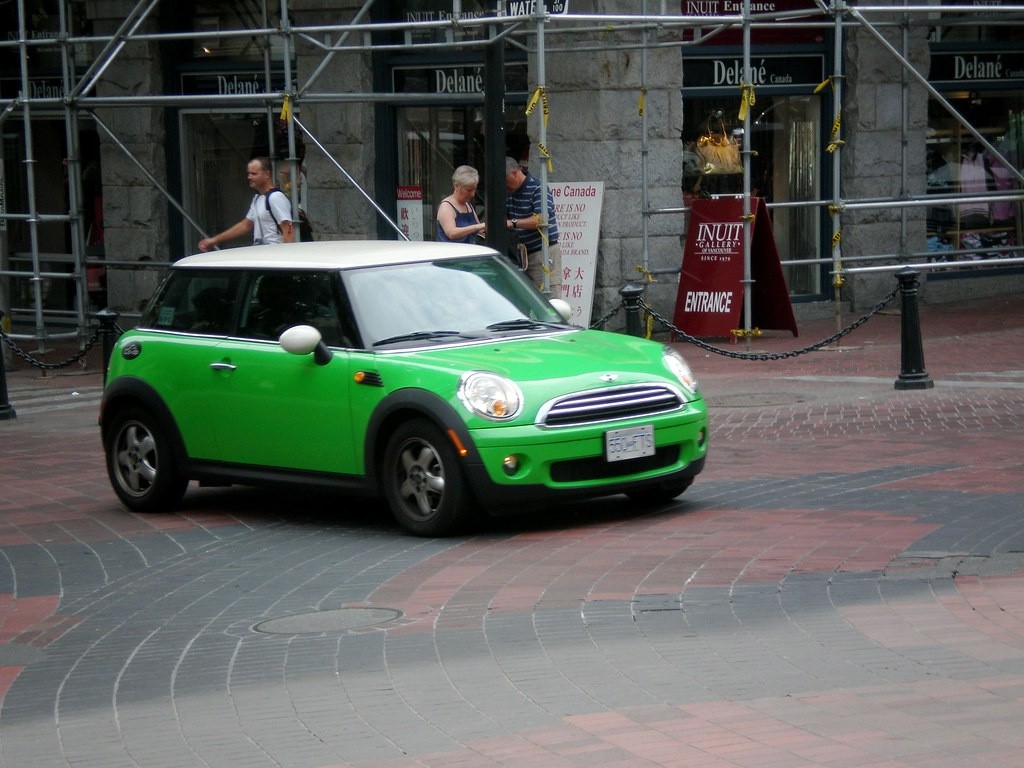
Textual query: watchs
512,218,517,228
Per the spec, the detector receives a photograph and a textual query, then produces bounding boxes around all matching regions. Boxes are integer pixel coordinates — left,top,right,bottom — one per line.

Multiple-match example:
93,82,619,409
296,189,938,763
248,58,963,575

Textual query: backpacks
254,188,313,242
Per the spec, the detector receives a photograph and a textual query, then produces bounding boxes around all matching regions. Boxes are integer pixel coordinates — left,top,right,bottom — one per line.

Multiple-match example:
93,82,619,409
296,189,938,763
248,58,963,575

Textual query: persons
199,158,313,252
436,165,485,272
505,154,562,320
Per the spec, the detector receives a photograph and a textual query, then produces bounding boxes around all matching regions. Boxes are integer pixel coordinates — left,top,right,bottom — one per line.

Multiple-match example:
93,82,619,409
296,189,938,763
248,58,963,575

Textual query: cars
107,237,701,531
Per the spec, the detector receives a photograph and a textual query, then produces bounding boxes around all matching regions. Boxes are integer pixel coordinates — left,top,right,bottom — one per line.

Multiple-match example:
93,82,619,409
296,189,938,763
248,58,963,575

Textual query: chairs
189,286,235,335
244,272,297,339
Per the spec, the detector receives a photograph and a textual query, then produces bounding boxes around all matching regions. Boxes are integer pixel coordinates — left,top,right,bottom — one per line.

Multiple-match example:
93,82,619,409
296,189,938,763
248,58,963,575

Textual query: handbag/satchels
696,113,744,174
